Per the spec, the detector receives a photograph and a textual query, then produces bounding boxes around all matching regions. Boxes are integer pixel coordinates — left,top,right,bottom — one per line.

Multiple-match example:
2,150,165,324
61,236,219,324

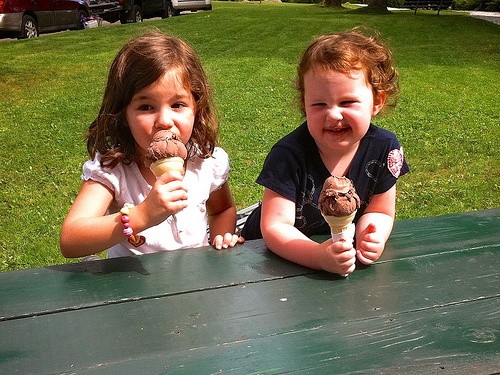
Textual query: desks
0,205,500,375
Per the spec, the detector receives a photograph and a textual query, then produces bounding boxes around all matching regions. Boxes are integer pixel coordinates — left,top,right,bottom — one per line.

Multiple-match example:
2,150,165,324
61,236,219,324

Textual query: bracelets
119,202,146,247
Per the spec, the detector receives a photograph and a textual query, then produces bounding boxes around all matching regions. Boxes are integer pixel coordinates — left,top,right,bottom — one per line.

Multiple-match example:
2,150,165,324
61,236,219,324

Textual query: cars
0,0,212,39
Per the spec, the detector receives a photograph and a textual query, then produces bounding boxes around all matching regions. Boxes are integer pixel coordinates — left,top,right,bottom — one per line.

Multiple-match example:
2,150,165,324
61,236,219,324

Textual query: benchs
402,5,448,15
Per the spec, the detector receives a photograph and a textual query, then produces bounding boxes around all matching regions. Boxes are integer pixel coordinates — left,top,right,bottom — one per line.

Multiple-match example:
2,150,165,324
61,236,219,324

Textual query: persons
238,25,411,278
60,29,245,258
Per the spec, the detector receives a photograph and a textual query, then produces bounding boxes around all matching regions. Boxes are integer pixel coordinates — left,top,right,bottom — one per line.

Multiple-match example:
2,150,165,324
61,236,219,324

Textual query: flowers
80,14,103,26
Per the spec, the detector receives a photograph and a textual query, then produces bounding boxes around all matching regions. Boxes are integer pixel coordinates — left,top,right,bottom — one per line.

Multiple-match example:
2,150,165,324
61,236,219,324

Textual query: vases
82,20,98,29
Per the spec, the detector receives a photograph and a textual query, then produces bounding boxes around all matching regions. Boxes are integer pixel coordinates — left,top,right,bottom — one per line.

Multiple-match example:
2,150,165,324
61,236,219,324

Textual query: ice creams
144,129,187,180
318,175,361,244
